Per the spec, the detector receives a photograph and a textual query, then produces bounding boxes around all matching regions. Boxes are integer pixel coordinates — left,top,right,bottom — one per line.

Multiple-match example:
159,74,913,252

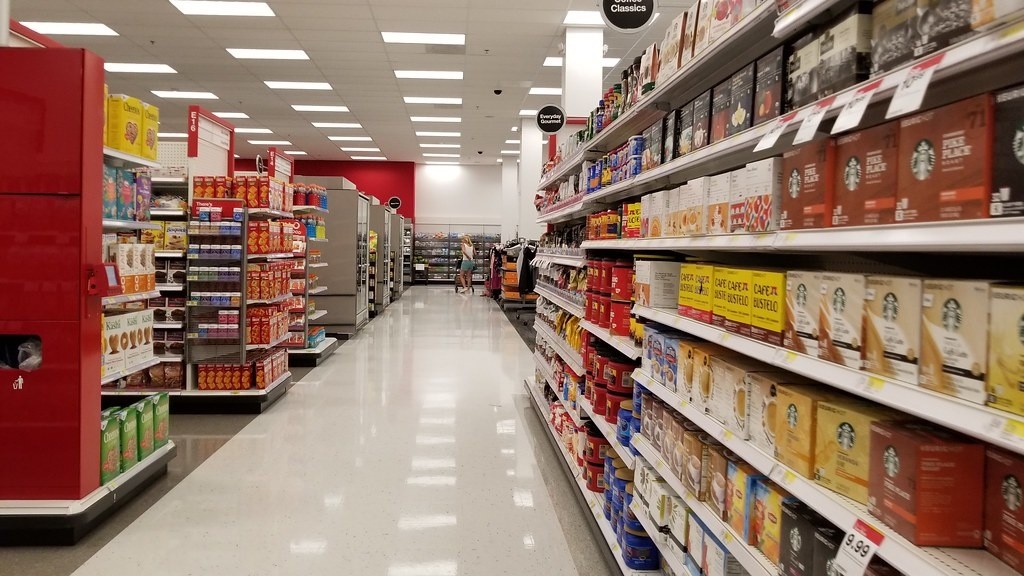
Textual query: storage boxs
103,83,160,160
103,232,171,486
194,178,293,389
634,0,1024,576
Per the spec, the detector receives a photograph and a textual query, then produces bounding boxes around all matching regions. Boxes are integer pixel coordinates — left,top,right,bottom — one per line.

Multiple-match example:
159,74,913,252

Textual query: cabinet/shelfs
287,204,339,366
449,238,483,282
575,104,669,576
523,148,608,482
102,146,176,505
413,238,449,281
101,177,294,414
403,224,414,281
631,0,1024,576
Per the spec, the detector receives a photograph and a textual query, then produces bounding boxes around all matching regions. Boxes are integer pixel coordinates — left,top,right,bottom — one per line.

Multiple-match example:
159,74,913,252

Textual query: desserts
104,231,185,356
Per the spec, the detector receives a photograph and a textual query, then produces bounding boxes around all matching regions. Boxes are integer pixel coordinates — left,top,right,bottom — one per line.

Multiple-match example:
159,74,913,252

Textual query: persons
459,235,477,293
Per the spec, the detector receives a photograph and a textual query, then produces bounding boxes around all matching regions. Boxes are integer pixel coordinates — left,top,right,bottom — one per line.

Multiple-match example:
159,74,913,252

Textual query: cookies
193,187,290,389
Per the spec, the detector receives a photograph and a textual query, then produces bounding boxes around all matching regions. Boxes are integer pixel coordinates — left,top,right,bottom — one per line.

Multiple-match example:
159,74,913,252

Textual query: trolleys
454,257,477,293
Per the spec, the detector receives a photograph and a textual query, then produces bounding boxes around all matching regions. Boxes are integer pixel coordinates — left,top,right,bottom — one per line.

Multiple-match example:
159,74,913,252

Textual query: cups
609,136,644,183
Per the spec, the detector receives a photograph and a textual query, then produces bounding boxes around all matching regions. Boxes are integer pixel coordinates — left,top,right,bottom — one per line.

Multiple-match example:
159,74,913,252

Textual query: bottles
294,213,325,239
189,207,244,236
585,198,643,240
586,153,611,194
198,310,240,337
308,273,319,290
584,336,652,457
309,249,321,264
582,420,661,570
191,291,242,306
188,244,242,258
308,298,316,316
584,254,675,348
594,47,662,133
188,266,241,282
293,183,328,209
308,326,325,348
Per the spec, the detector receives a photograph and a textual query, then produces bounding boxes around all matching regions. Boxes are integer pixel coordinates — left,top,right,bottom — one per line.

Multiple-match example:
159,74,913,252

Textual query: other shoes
460,289,471,293
